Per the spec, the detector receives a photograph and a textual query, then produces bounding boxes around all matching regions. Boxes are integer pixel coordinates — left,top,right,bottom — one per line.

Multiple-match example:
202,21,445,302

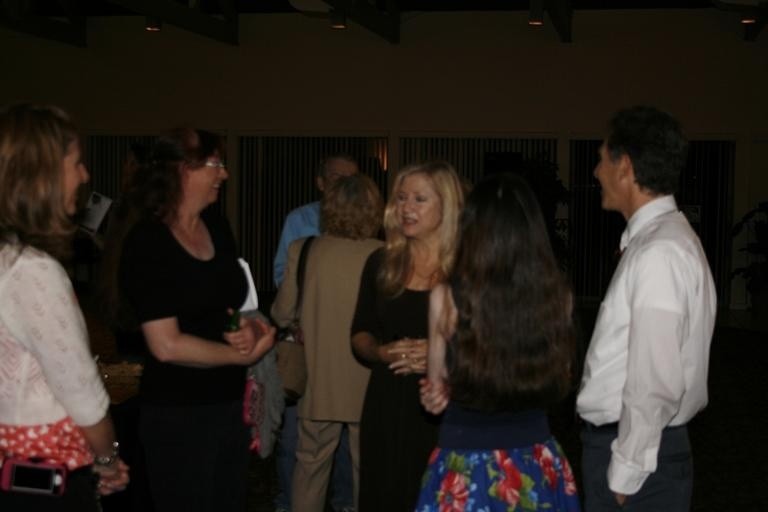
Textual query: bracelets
90,441,119,466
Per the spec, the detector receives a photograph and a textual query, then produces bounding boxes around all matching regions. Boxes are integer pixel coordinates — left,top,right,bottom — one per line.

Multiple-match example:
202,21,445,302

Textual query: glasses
204,160,227,168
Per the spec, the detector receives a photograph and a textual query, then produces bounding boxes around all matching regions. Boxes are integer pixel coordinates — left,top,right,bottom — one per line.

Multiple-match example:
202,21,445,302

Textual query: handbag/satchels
275,334,305,406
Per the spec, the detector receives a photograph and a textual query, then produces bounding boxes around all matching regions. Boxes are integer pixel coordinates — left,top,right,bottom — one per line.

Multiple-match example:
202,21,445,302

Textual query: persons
269,172,389,511
577,104,720,510
1,103,135,510
270,153,367,510
414,174,583,509
350,158,473,511
118,123,277,510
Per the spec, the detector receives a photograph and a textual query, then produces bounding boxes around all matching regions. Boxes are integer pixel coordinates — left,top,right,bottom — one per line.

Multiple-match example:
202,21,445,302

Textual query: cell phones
0,456,67,498
